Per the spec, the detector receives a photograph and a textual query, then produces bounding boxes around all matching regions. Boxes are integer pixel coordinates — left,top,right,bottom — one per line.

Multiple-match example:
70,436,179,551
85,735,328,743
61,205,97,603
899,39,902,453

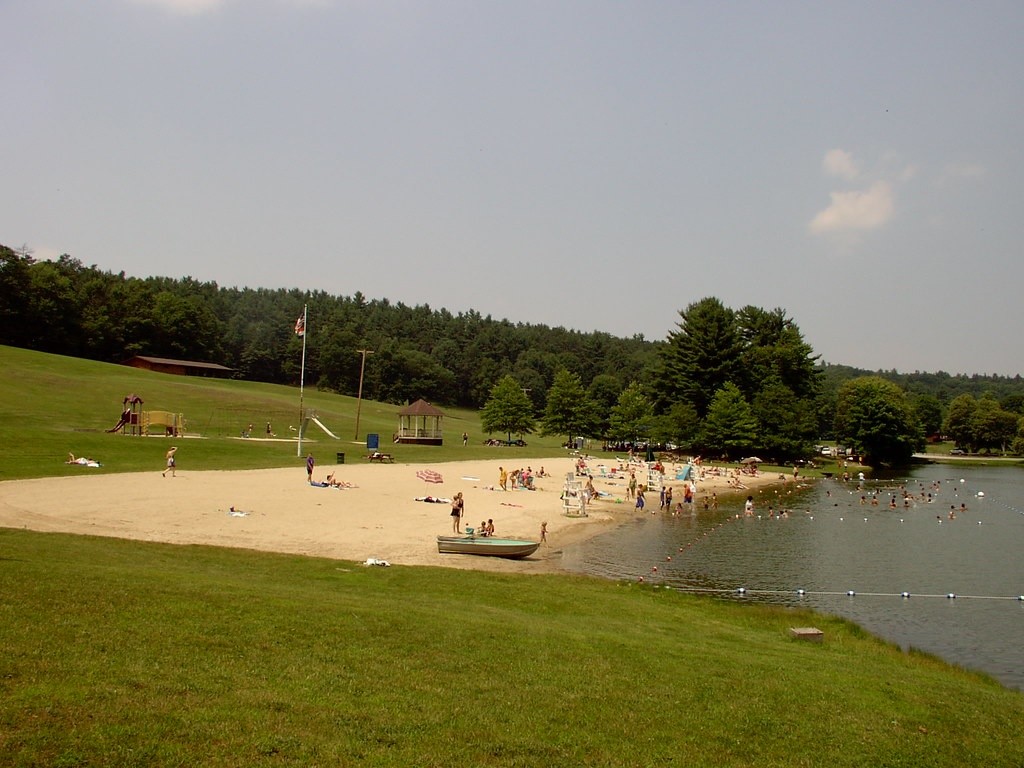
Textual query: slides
312,417,340,440
104,420,126,432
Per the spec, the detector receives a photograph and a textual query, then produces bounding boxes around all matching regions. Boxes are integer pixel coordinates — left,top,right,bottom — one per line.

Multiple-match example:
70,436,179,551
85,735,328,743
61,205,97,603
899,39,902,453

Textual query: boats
437,533,541,559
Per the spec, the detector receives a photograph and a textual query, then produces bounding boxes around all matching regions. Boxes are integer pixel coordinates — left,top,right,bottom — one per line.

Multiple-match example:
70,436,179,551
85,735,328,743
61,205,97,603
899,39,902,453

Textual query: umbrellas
741,456,762,472
416,469,444,498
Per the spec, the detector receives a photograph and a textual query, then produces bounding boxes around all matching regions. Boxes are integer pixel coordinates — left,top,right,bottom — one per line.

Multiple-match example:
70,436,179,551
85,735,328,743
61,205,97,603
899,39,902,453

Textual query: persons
162,447,178,478
450,491,464,535
498,448,971,522
63,452,101,465
462,432,468,446
306,452,313,484
248,425,254,438
266,420,272,439
240,429,245,438
479,518,494,535
540,521,547,542
230,506,235,512
326,470,347,487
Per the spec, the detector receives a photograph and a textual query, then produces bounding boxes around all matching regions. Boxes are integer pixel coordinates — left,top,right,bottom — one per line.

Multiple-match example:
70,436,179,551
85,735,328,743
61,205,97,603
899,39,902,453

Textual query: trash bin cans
337,452,345,464
